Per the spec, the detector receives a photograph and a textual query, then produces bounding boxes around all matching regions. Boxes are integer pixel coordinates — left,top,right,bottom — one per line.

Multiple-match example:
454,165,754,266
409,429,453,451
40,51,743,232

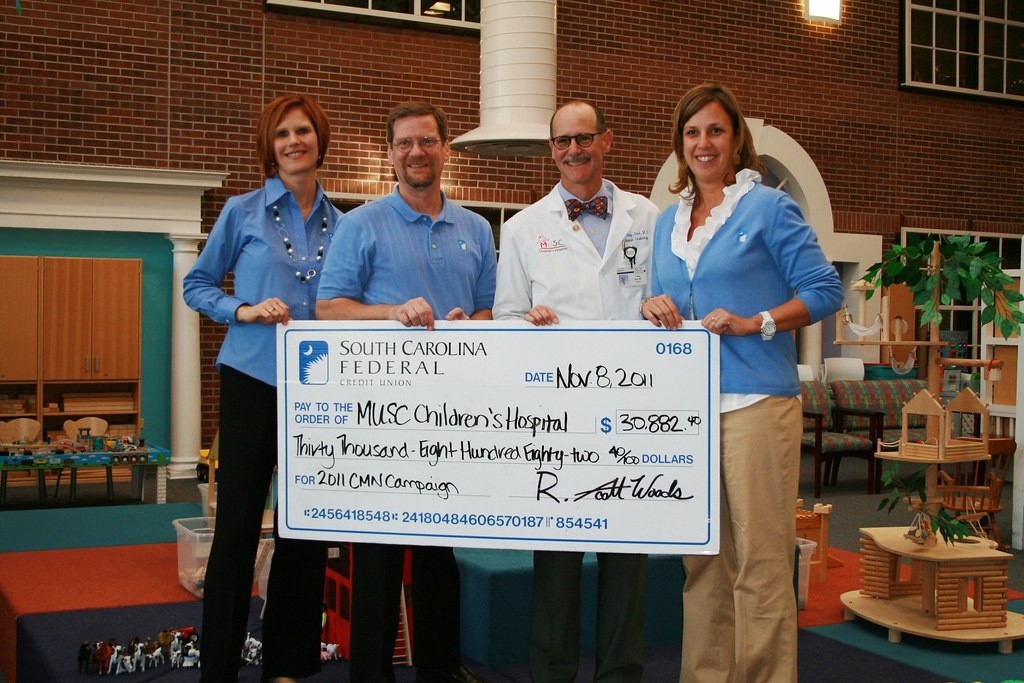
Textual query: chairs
52,418,108,497
935,438,1016,551
0,418,44,500
800,380,878,498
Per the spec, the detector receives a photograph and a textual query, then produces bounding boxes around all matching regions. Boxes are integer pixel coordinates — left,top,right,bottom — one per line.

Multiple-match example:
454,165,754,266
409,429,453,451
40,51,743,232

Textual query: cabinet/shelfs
0,255,141,486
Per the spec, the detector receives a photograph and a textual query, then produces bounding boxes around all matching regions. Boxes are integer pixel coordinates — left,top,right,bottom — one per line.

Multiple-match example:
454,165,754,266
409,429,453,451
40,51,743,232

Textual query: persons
311,100,497,683
488,101,661,683
184,92,346,683
638,84,844,683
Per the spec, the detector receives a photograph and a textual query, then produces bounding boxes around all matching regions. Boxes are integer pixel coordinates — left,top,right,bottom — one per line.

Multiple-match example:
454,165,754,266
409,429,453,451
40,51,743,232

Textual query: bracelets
640,294,659,315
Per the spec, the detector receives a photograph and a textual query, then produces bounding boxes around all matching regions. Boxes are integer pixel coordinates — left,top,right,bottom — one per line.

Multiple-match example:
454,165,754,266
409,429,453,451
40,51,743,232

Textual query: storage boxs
172,517,215,597
197,483,218,517
795,537,817,609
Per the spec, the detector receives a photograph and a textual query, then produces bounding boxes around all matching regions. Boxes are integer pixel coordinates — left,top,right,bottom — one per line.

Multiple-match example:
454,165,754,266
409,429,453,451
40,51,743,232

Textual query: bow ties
564,196,608,222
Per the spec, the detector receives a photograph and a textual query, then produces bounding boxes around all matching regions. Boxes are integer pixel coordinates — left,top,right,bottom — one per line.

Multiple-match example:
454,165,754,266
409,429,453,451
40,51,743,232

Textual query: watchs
759,310,776,341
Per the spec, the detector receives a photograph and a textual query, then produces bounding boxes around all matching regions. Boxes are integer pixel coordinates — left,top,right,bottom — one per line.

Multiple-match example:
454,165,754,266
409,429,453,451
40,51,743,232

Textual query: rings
711,316,718,322
268,307,274,313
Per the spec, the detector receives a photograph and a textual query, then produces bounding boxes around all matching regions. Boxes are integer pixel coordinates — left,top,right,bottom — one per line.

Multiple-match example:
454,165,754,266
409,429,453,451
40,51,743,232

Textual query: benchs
831,379,929,492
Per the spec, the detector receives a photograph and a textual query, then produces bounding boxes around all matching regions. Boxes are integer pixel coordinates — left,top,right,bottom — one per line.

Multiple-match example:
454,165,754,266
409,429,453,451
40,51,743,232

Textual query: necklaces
273,196,327,284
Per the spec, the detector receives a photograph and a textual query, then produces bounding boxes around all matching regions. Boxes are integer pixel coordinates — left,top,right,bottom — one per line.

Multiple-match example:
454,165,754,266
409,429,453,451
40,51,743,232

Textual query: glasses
391,136,443,153
551,128,604,150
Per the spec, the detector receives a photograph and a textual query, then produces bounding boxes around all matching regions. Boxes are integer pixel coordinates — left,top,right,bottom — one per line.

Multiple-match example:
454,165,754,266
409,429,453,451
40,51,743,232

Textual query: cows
321,642,340,661
129,627,200,672
242,632,262,666
77,640,133,676
